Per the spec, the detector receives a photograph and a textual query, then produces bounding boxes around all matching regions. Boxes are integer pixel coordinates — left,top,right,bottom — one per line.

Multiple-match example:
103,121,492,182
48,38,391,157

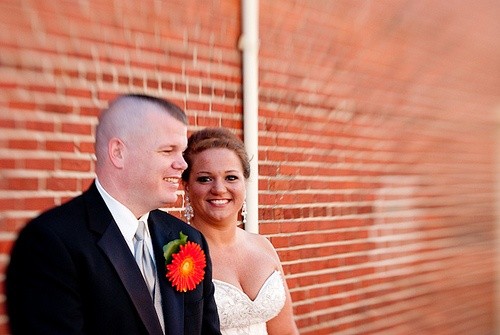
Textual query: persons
3,94,227,335
179,127,300,335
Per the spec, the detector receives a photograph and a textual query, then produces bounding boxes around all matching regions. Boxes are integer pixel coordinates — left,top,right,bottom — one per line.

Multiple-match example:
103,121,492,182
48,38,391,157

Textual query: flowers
163,232,206,292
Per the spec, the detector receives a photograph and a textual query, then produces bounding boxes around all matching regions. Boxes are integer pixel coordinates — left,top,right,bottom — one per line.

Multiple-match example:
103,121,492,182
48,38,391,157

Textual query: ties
134,221,158,311
